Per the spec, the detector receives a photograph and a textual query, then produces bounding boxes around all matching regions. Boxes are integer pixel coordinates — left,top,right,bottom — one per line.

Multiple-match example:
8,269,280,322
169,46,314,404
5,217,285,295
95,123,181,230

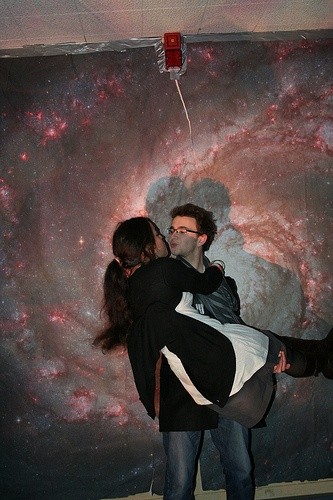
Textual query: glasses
167,227,201,235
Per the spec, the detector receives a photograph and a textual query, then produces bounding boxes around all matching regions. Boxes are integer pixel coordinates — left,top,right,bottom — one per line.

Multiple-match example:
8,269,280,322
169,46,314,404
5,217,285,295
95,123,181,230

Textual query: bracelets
210,259,225,268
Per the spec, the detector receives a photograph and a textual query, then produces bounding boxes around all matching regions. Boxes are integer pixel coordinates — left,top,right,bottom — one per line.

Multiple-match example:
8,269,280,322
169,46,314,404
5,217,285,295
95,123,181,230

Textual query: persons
91,216,333,381
155,203,290,500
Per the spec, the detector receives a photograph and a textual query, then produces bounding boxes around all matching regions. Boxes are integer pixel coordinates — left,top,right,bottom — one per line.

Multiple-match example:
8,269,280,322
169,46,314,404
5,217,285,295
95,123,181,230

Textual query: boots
303,349,333,380
296,328,333,352
